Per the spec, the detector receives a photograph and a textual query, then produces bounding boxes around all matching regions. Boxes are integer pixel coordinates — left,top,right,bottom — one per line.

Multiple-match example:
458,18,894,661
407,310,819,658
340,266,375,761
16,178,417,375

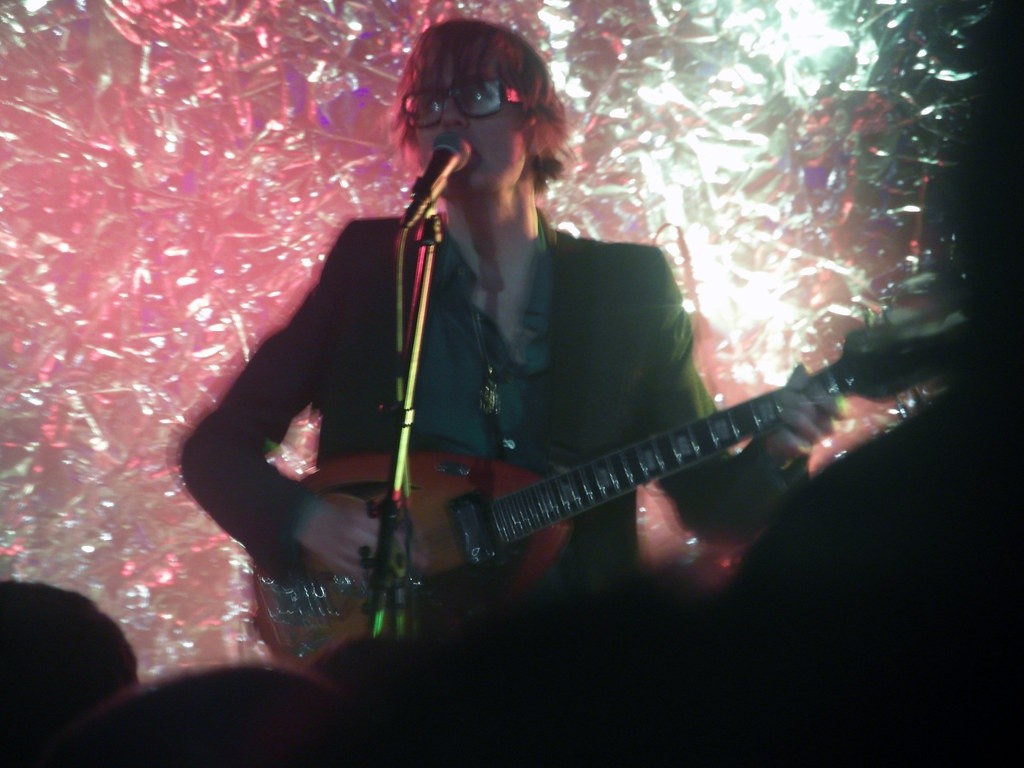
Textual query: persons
174,19,847,640
0,374,1024,768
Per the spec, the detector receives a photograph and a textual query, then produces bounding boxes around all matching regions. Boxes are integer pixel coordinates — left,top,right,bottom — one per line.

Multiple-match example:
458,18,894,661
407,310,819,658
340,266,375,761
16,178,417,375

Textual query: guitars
248,300,974,673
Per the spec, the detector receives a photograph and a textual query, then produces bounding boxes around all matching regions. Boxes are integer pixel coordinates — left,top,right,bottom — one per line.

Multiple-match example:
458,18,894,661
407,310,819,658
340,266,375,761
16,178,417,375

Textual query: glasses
402,78,521,129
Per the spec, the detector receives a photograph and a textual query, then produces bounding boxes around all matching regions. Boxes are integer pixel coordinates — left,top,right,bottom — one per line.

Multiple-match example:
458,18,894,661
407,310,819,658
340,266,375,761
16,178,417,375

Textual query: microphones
401,131,473,229
